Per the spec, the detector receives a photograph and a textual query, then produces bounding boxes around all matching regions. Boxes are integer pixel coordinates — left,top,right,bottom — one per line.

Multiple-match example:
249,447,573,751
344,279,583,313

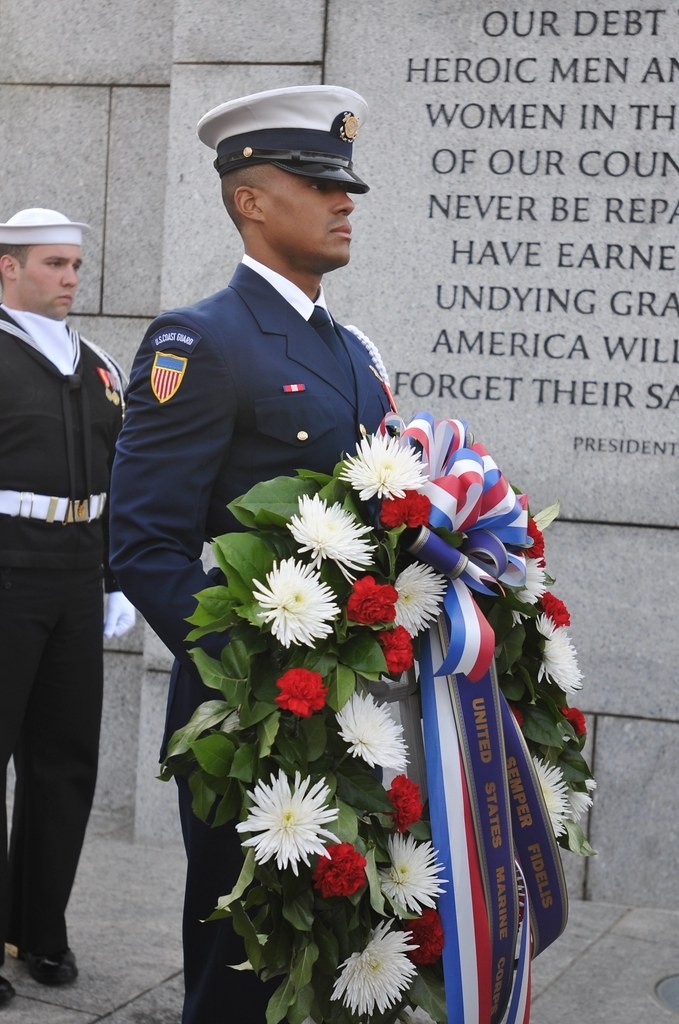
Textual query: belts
0,490,106,526
209,544,221,567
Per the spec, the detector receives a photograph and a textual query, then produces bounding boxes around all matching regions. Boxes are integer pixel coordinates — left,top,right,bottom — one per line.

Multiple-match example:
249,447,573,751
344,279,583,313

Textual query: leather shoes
0,977,16,1000
5,939,78,985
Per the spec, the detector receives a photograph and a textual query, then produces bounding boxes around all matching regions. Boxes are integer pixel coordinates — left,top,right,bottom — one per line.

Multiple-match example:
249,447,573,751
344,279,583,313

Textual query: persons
0,208,146,1006
107,83,404,1024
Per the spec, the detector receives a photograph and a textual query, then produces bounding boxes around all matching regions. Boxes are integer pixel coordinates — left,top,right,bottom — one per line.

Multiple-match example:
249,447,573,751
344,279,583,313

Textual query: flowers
150,412,597,1024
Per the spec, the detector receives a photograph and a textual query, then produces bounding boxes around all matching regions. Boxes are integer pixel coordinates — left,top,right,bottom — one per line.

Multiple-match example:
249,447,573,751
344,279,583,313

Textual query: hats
196,85,370,194
0,208,89,247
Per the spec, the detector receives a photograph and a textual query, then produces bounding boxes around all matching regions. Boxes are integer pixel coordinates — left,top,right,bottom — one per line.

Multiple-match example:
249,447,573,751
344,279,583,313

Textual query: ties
310,306,357,394
